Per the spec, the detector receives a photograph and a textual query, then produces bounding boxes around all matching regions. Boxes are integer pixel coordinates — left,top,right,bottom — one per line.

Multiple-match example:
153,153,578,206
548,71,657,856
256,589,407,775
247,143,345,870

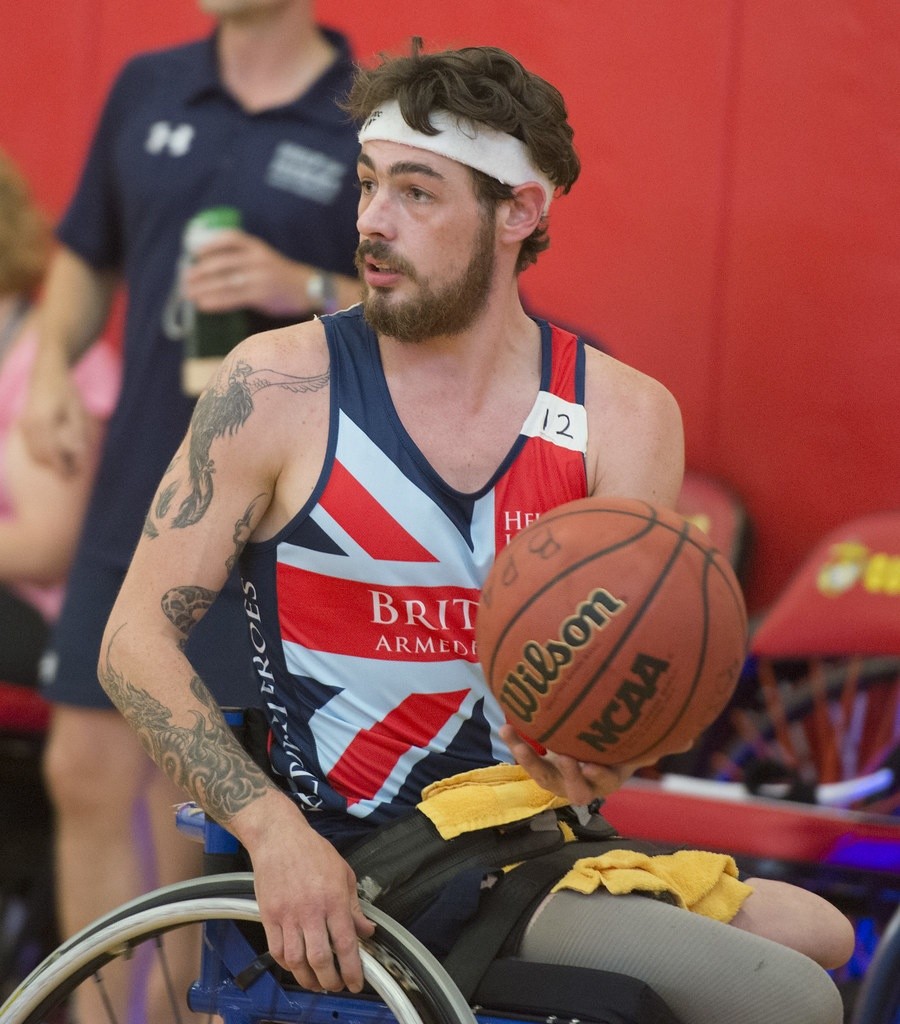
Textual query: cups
178,207,248,397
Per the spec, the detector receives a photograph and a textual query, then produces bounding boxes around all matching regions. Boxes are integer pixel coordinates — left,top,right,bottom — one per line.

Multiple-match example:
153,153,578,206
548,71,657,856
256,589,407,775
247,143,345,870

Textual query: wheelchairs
1,706,900,1023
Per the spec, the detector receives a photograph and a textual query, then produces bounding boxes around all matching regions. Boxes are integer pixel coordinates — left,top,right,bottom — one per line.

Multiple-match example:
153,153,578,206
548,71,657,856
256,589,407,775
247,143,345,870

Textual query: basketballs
467,489,754,771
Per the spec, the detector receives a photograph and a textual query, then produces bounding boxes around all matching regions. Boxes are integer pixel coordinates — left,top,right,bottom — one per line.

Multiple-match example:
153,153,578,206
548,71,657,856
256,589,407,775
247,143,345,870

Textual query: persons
96,42,855,1023
30,0,374,1024
2,173,116,1022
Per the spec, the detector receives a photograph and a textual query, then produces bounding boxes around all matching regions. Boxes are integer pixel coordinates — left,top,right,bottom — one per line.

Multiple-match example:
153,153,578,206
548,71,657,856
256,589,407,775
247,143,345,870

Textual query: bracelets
308,266,335,316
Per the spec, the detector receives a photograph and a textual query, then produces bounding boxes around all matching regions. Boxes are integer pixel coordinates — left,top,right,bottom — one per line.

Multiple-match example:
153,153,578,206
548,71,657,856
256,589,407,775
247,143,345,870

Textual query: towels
412,763,756,925
